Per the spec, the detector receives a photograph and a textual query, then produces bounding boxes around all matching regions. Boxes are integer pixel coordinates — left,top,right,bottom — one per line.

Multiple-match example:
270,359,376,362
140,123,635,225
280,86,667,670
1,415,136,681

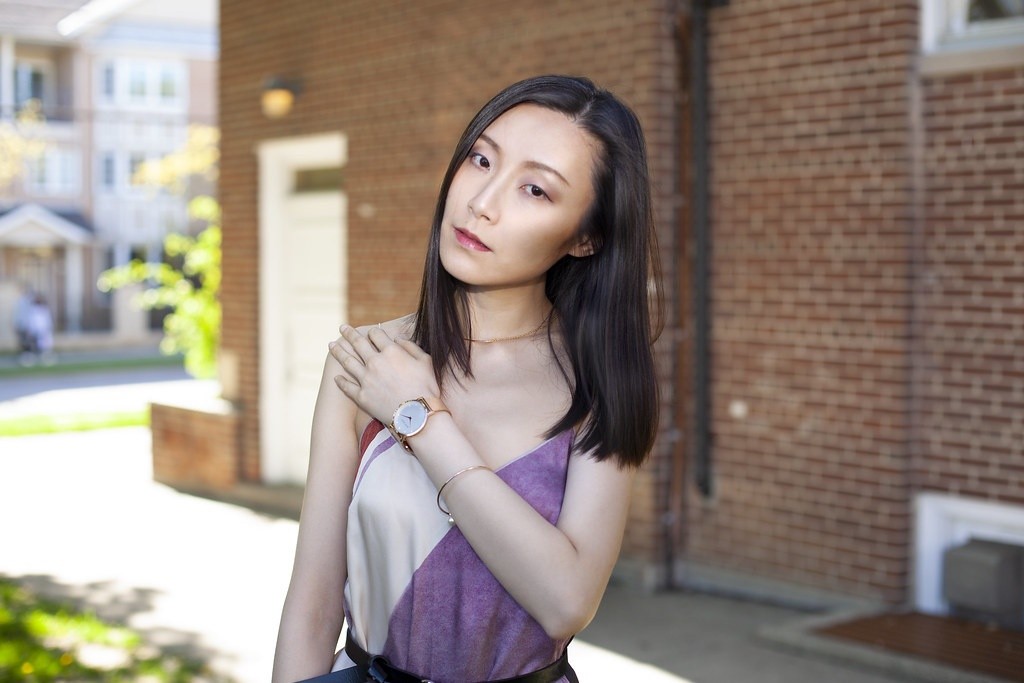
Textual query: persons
12,287,56,367
272,75,665,682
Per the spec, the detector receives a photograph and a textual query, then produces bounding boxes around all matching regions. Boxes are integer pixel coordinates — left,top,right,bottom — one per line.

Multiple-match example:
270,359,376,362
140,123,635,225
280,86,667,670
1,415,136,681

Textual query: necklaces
465,325,544,343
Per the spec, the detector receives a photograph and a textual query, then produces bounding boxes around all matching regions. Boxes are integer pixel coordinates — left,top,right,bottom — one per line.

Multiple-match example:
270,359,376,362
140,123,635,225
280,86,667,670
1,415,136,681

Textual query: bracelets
436,465,495,524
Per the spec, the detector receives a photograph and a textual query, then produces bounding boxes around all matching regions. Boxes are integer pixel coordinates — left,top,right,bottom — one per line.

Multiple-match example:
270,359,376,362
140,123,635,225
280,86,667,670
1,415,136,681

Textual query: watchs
389,396,453,457
